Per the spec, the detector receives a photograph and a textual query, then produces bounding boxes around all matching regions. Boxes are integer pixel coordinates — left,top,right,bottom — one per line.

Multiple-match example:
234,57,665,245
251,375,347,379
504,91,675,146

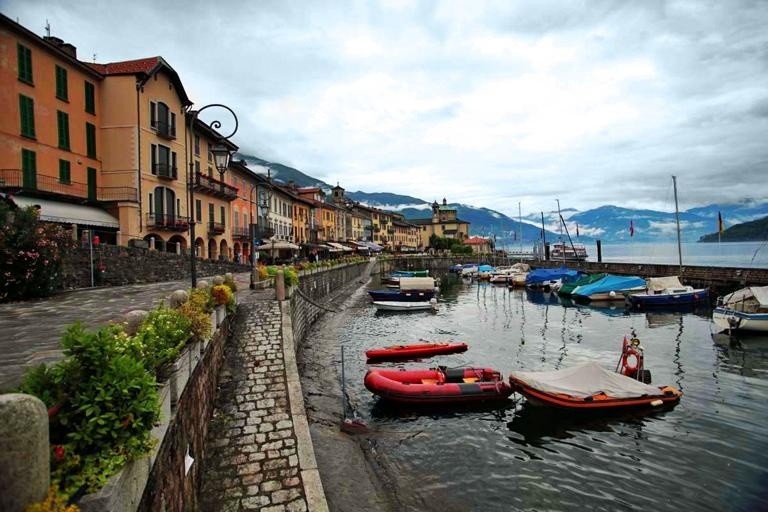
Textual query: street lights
189,100,237,292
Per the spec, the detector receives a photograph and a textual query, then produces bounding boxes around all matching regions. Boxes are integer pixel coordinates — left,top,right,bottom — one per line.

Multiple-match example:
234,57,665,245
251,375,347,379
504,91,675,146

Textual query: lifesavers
623,349,641,373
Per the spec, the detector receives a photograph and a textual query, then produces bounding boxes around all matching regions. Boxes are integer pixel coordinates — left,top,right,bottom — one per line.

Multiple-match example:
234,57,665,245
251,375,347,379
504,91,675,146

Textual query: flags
718,210,726,234
629,220,635,237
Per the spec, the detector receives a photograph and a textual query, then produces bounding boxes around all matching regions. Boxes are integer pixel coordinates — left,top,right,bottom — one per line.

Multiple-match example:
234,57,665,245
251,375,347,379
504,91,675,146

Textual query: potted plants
0,281,236,512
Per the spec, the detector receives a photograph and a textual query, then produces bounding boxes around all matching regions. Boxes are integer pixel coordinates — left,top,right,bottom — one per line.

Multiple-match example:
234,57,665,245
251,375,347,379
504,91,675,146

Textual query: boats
364,365,513,404
450,263,705,305
713,286,767,330
367,270,442,312
365,342,468,360
507,329,683,413
